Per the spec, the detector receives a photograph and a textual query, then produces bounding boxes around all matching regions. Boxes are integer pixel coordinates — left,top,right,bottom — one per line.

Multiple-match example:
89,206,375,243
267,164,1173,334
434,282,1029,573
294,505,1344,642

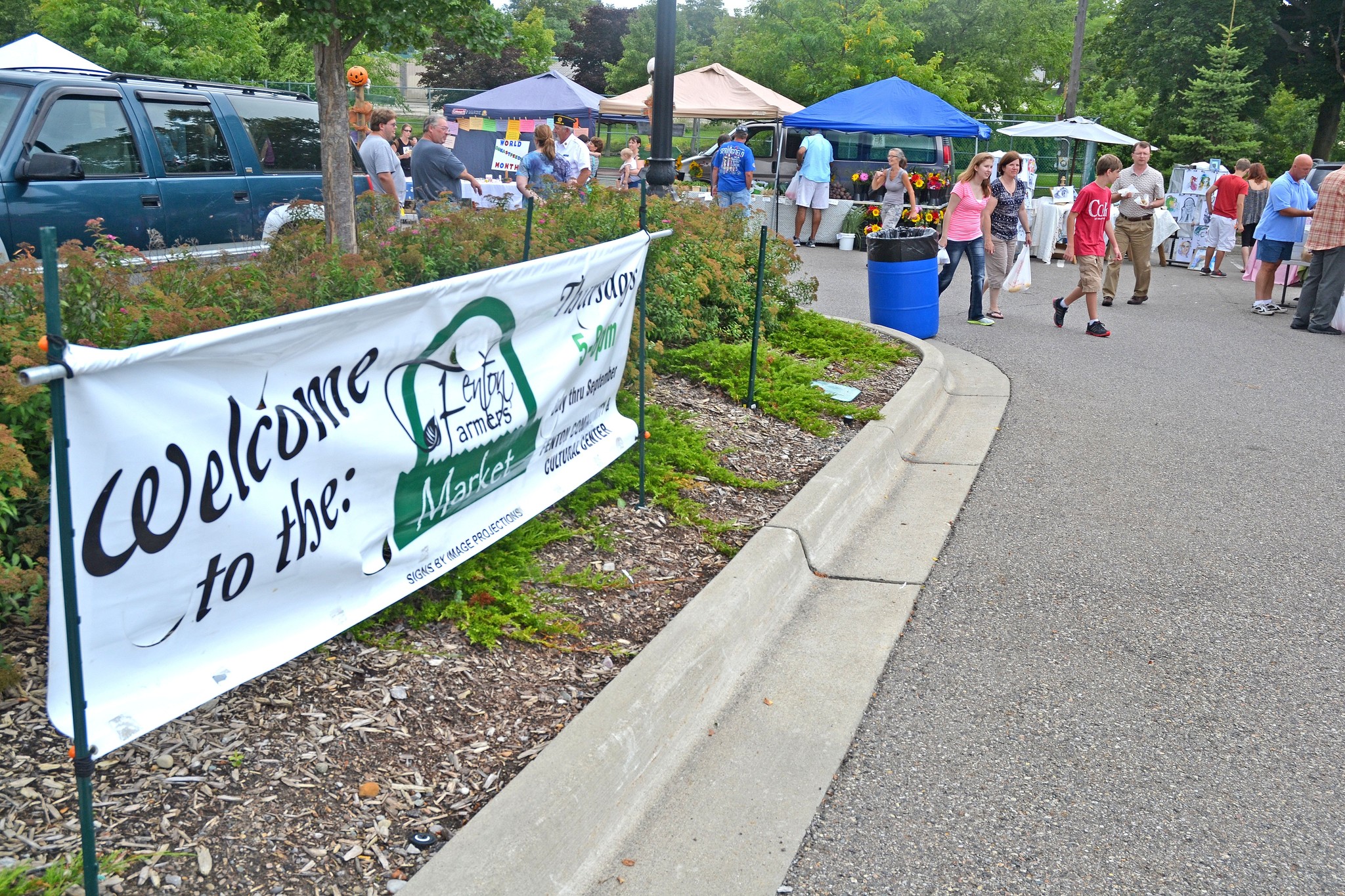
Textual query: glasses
386,123,397,127
434,125,451,132
736,127,747,131
887,154,897,159
1132,152,1151,157
403,129,412,133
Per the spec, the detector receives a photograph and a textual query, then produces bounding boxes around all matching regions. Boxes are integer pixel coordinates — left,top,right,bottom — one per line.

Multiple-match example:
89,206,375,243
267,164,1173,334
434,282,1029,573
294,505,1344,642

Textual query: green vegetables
754,182,764,194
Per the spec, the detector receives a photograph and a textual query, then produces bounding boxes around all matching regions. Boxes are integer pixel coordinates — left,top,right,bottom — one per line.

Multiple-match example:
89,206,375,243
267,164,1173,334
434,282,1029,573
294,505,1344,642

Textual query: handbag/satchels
937,245,950,265
1002,242,1033,293
784,168,800,200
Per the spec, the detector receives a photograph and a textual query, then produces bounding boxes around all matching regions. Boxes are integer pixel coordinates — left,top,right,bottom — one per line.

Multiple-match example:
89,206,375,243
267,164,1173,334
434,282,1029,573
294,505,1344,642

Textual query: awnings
596,63,808,233
771,75,993,244
444,69,652,178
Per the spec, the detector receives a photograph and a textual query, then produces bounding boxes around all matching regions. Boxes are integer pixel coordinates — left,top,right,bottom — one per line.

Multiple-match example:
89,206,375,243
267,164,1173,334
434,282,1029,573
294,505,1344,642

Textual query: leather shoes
1102,297,1112,305
1127,295,1148,304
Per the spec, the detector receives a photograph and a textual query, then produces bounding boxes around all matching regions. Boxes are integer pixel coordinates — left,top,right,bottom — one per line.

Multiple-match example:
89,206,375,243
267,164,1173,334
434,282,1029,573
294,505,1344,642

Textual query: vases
850,167,952,208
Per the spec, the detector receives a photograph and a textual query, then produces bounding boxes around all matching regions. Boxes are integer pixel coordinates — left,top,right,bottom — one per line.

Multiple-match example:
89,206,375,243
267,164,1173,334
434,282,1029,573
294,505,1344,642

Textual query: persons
1251,154,1317,315
619,134,646,189
515,124,577,210
793,128,834,248
358,107,407,233
153,106,275,173
1199,158,1250,277
553,113,591,190
1241,163,1272,273
410,114,483,225
391,123,418,177
710,125,756,217
1290,163,1345,335
1053,154,1122,337
578,134,603,177
871,148,918,231
982,150,1032,319
1102,141,1165,306
938,152,995,326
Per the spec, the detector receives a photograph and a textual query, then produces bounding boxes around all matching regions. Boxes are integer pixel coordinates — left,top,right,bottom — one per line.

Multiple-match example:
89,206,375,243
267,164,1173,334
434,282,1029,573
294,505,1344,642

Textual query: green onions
761,180,775,196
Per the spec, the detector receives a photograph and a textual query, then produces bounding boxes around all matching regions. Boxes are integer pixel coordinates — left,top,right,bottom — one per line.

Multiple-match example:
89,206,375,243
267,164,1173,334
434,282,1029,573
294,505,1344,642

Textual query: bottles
499,174,502,182
489,173,492,181
496,177,498,180
485,173,489,179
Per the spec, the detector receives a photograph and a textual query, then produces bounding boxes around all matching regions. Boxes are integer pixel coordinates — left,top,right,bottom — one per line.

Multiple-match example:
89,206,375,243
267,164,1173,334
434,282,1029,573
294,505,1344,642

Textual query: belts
1119,212,1153,222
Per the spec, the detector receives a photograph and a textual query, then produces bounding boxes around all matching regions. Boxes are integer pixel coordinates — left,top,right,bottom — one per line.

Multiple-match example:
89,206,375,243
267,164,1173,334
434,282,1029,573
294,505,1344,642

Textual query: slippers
986,311,1004,318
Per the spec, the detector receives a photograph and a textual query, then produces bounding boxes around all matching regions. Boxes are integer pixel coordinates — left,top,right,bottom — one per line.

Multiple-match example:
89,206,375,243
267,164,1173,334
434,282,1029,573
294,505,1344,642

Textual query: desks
1032,196,1183,267
461,177,527,210
688,193,950,249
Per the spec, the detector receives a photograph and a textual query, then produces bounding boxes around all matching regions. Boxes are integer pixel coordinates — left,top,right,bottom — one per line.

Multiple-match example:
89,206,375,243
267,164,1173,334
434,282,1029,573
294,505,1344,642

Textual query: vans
674,120,955,202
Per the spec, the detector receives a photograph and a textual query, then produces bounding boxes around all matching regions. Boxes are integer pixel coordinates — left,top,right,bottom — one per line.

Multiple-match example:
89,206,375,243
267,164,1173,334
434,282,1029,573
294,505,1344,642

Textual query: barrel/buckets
839,232,856,250
852,182,947,235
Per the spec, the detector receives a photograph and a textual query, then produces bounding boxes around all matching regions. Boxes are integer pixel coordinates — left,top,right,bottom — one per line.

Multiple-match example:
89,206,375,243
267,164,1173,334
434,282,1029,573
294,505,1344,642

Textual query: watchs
1025,231,1031,233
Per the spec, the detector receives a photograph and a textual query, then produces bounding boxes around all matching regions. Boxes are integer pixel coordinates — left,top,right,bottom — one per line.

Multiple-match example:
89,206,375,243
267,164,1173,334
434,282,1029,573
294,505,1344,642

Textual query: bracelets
1149,203,1154,209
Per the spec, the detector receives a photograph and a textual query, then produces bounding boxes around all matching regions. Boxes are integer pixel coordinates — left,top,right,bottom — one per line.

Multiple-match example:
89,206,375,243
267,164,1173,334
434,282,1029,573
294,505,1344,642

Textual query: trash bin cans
865,226,940,340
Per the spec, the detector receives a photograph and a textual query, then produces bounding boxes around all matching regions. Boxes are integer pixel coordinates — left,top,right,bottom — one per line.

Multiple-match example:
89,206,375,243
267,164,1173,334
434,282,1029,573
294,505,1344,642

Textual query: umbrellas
997,116,1160,188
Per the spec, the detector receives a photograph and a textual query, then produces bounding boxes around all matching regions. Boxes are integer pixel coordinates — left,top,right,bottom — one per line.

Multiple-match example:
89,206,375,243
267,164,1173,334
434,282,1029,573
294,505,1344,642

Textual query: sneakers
1053,297,1068,327
1291,322,1311,329
805,236,816,247
1308,324,1342,335
1200,267,1212,275
1265,301,1288,313
967,317,995,325
1086,321,1110,337
792,238,801,246
1251,302,1275,316
1210,269,1227,277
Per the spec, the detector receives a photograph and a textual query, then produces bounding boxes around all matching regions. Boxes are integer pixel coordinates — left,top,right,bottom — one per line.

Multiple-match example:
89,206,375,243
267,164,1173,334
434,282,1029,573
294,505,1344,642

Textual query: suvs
0,66,376,268
1304,157,1345,228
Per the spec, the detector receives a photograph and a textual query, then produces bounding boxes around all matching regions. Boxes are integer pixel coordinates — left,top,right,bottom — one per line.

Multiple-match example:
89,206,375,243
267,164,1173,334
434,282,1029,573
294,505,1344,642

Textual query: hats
553,112,577,128
736,124,748,133
